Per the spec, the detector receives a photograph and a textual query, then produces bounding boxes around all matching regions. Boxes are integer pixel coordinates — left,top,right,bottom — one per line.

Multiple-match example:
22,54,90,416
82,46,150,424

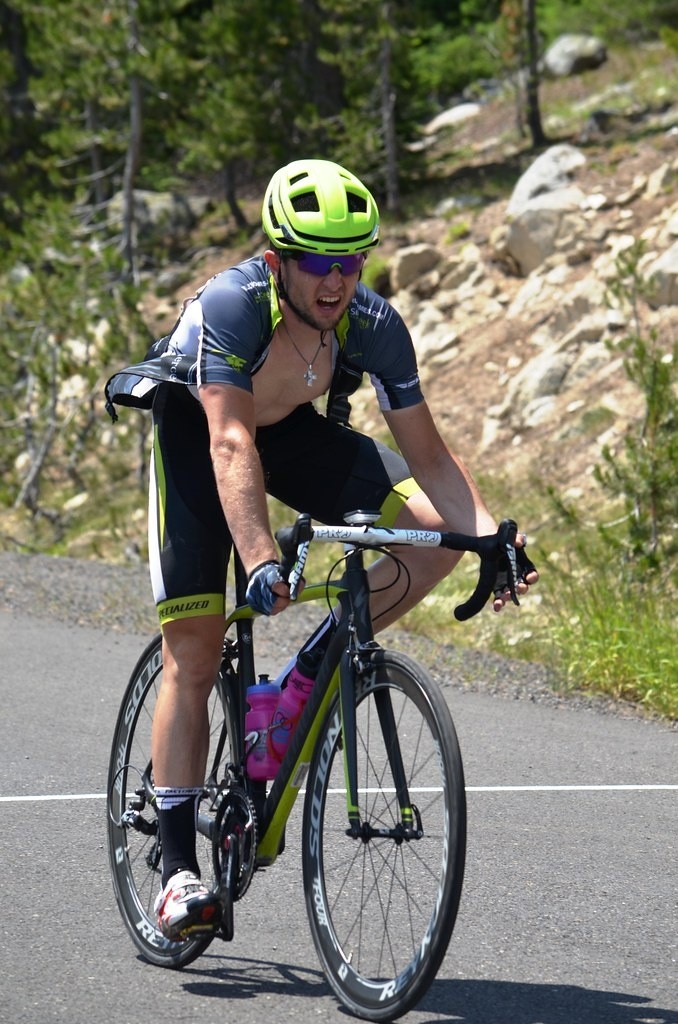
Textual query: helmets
261,158,382,258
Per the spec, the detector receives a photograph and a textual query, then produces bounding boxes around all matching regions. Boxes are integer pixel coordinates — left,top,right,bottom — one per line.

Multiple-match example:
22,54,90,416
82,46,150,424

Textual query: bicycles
107,514,526,1023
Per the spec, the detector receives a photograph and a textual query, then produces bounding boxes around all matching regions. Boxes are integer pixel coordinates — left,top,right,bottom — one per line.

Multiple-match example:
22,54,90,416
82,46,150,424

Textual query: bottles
246,672,281,781
265,653,320,757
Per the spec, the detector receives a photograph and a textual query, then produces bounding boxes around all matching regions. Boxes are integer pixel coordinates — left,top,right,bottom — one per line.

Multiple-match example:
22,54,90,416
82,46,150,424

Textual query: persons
107,160,539,939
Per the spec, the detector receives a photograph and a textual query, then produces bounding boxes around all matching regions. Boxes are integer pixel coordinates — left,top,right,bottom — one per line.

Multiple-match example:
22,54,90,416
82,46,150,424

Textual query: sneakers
153,870,224,945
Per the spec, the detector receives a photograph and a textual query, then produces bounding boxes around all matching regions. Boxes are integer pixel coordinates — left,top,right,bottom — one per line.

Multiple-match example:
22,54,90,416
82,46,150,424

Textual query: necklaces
279,304,327,388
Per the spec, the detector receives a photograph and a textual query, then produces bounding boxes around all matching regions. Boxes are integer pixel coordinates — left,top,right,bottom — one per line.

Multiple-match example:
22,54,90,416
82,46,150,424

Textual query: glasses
270,247,370,276
